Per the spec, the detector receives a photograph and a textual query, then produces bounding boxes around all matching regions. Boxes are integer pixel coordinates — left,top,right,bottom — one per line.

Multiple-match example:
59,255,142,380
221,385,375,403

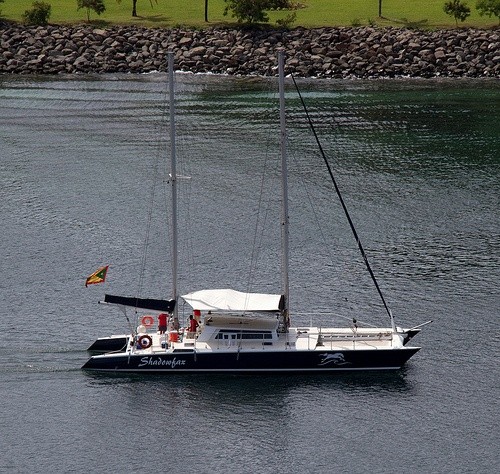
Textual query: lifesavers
138,335,152,348
141,315,154,328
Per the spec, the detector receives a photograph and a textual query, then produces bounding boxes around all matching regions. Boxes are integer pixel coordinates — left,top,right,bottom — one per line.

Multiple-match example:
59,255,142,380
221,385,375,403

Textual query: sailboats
80,48,432,378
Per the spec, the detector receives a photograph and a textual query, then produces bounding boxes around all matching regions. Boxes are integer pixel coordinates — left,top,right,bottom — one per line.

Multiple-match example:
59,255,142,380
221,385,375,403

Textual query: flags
85,265,109,287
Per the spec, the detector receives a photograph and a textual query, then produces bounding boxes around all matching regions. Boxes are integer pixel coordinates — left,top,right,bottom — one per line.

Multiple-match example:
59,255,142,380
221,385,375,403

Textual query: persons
158,312,170,334
187,315,200,333
